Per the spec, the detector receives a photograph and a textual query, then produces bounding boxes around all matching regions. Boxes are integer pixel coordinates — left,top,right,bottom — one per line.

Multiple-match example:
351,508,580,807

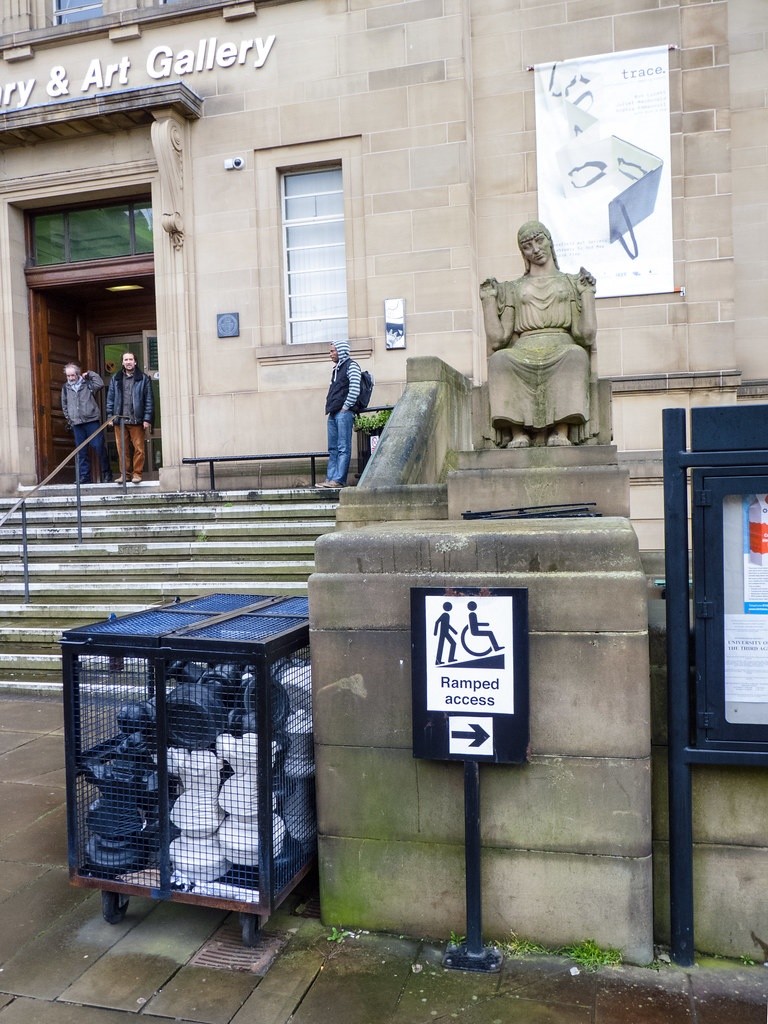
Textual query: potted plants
354,410,392,478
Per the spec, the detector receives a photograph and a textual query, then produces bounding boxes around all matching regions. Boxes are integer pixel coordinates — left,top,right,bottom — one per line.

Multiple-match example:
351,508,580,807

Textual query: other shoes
114,473,142,482
315,480,343,488
103,473,112,483
74,476,90,484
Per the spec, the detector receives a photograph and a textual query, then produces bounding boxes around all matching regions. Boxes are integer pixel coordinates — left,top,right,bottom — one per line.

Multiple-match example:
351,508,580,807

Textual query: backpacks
344,360,375,411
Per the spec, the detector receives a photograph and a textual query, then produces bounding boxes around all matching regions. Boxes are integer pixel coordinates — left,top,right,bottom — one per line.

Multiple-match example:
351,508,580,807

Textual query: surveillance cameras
233,158,244,169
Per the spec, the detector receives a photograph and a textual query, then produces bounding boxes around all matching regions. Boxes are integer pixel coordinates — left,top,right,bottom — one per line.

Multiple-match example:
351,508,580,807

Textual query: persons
477,221,598,449
61,363,113,485
106,351,155,483
314,338,362,489
